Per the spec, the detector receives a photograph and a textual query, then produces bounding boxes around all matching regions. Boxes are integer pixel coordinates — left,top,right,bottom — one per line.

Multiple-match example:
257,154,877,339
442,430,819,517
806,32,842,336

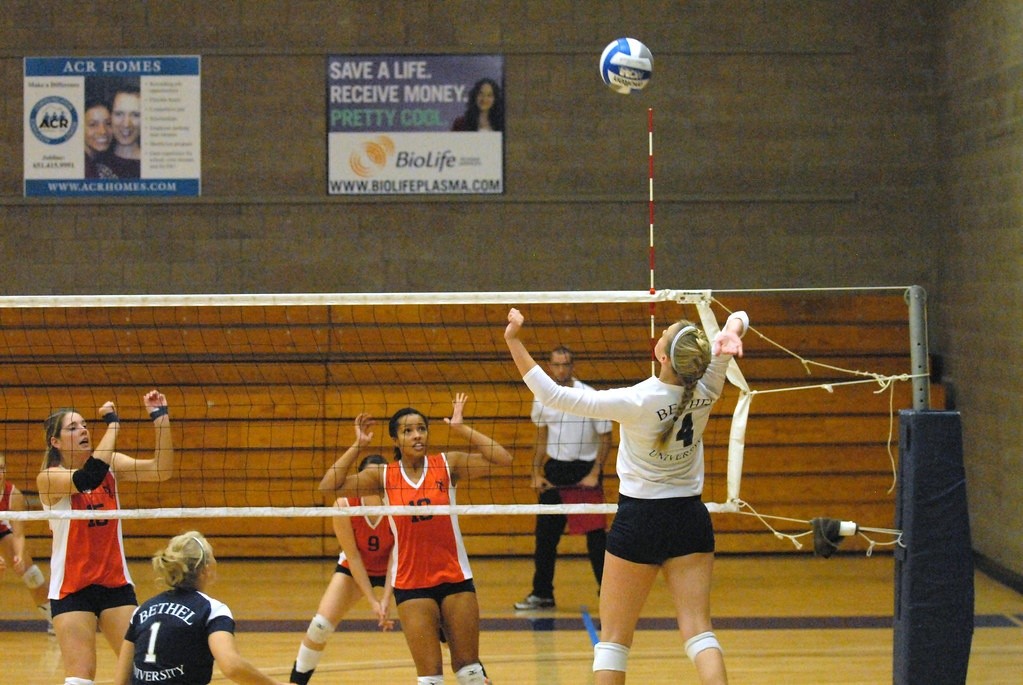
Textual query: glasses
551,361,572,368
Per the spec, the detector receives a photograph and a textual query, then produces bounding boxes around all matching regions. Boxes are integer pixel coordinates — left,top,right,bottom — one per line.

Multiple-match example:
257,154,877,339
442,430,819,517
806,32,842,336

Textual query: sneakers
513,593,556,611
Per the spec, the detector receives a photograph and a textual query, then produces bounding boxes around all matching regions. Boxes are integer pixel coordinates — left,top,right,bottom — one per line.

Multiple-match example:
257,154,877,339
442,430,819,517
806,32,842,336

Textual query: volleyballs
600,37,654,94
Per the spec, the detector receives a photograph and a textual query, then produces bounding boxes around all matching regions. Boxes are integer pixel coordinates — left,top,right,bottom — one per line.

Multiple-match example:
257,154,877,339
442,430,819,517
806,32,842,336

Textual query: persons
451,77,503,133
317,392,513,685
512,345,614,612
289,453,452,685
503,306,751,685
113,530,297,685
92,85,141,178
35,390,175,685
85,98,116,179
0,450,59,637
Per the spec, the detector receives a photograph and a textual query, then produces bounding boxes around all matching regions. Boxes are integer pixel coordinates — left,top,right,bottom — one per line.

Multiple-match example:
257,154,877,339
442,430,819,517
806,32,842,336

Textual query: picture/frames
324,50,510,199
18,53,204,200
